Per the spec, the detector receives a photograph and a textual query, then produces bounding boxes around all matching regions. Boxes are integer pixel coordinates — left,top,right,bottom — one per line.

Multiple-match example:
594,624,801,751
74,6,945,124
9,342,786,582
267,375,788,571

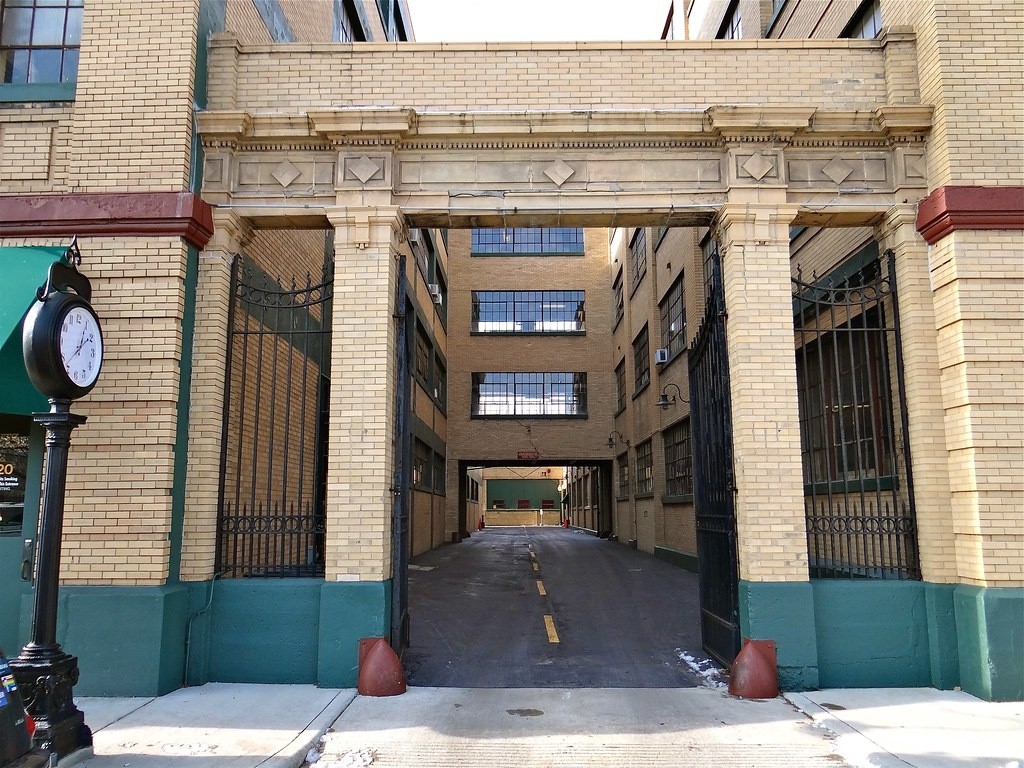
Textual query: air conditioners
429,284,439,299
410,229,419,246
434,293,442,306
653,349,667,365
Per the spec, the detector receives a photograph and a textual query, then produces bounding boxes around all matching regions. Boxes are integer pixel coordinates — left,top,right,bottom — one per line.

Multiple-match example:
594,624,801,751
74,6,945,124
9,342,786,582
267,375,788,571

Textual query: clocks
23,292,105,397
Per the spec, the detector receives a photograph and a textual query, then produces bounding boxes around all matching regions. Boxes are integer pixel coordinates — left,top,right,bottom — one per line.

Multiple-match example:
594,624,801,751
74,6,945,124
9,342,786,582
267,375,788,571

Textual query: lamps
606,431,630,448
655,383,689,410
